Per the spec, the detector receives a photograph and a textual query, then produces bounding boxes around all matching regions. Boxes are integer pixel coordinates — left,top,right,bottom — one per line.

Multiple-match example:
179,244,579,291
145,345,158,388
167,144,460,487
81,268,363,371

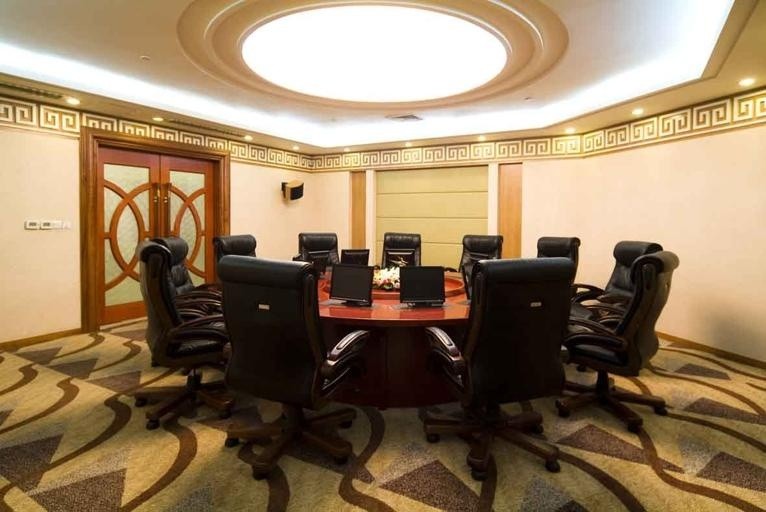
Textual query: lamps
237,1,515,111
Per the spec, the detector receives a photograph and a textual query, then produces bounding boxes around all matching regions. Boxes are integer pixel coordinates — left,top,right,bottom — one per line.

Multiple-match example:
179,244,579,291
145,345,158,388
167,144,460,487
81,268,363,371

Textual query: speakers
285,179,305,202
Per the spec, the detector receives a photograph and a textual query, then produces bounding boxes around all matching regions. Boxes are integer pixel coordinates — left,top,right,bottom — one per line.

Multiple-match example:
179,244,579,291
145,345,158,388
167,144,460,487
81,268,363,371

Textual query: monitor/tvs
470,253,489,265
400,266,445,307
462,264,475,300
307,251,330,272
341,250,369,265
329,263,374,307
385,249,415,268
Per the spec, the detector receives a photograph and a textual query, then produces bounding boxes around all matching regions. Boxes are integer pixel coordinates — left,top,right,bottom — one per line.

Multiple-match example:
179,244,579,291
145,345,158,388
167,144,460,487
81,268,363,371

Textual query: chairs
563,241,663,374
459,234,503,272
219,253,358,482
134,244,230,431
297,233,341,268
555,251,680,431
416,255,578,479
536,236,580,281
211,236,257,258
147,234,229,371
381,232,422,266
